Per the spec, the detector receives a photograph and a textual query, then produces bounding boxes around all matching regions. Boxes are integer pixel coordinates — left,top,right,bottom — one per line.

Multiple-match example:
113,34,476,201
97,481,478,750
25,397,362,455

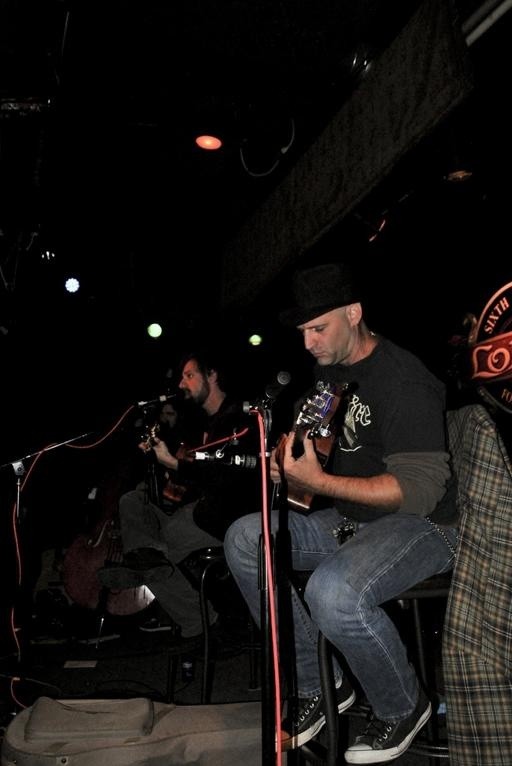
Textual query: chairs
314,404,502,765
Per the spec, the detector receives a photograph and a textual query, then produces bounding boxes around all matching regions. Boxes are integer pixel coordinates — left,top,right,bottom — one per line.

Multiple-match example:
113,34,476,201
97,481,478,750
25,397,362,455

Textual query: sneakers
193,617,221,663
344,690,435,765
96,558,175,590
138,618,172,632
274,675,358,752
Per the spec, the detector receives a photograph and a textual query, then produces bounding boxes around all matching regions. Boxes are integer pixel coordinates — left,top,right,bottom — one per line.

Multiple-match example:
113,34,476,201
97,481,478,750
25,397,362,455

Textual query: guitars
269,380,351,514
141,422,186,511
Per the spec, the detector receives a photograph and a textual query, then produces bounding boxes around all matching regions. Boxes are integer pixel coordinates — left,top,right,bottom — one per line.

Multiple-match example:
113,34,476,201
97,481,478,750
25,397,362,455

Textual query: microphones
259,370,292,410
135,391,178,410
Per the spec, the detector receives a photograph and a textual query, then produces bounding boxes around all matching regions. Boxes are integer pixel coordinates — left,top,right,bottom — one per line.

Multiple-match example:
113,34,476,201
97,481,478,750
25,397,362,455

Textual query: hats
279,261,380,326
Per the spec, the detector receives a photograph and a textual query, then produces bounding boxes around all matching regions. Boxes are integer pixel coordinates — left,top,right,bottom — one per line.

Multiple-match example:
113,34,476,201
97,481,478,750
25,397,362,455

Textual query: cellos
60,477,156,616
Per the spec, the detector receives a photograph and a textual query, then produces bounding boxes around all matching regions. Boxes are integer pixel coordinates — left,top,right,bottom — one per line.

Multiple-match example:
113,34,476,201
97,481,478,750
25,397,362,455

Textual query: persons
224,263,461,766
97,355,263,652
